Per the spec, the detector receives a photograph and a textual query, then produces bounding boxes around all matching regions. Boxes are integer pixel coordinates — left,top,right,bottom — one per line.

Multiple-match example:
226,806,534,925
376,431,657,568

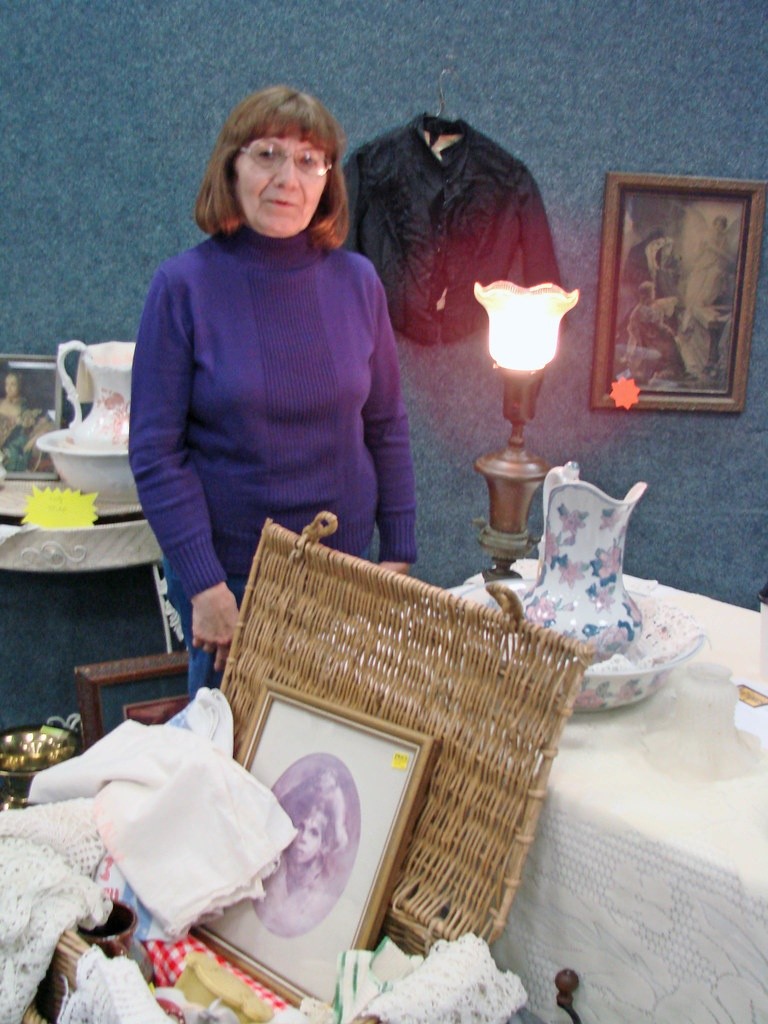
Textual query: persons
128,85,419,700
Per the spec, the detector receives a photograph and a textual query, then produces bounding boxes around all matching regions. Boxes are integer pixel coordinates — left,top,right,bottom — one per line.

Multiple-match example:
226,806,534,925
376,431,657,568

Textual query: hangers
426,66,459,149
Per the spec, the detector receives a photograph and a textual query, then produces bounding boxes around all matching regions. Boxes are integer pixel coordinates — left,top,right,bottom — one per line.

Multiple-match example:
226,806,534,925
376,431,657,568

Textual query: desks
287,557,767,1024
0,485,173,654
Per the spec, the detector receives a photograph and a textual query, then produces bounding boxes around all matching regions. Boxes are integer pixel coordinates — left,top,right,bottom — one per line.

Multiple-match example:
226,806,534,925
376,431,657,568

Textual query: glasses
240,139,332,178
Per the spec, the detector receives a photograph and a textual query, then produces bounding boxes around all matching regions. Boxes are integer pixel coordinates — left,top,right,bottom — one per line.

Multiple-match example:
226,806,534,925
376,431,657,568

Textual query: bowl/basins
36,426,142,503
462,578,703,712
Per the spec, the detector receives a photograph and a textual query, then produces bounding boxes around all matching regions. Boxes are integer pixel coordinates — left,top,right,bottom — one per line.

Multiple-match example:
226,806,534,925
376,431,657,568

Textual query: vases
75,646,189,752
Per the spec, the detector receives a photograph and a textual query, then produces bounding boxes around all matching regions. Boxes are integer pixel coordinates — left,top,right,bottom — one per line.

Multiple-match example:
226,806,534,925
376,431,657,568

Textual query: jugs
523,461,646,664
56,339,136,449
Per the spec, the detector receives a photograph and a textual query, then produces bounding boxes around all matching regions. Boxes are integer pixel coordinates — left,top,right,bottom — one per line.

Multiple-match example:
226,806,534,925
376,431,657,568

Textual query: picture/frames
589,168,768,414
189,678,443,1011
0,355,67,482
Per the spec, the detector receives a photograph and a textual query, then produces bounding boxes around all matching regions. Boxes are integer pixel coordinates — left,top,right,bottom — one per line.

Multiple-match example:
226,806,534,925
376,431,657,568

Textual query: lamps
473,280,580,582
